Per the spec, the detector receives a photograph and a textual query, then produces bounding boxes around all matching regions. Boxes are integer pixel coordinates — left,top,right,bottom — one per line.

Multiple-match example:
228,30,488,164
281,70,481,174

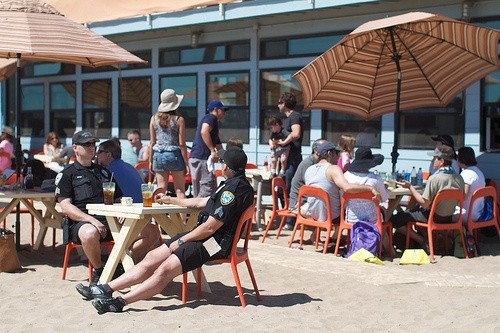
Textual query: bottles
401,170,405,179
26,167,33,190
410,167,417,186
417,168,423,186
264,156,268,171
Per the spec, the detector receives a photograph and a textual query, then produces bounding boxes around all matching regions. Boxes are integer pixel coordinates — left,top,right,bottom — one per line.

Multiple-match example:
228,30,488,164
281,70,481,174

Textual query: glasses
278,101,283,104
51,136,57,140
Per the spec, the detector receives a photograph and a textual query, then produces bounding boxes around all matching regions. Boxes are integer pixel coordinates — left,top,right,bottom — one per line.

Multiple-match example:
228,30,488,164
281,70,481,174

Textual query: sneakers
91,270,100,282
111,264,125,280
423,238,431,255
76,283,112,299
92,297,122,313
282,224,293,230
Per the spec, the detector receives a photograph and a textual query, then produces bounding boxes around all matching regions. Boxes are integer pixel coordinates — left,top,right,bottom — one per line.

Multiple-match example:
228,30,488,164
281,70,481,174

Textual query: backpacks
344,221,381,258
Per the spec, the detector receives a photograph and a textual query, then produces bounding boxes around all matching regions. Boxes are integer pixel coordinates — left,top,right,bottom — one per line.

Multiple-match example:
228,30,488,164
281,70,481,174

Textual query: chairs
0,148,500,308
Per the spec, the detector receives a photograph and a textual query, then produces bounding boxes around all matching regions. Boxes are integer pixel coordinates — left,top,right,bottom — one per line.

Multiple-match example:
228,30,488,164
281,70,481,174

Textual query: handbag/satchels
0,228,21,273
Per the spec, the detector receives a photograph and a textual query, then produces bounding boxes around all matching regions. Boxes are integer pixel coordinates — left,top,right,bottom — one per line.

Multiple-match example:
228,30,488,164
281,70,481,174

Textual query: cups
103,182,115,205
122,197,133,206
141,184,154,207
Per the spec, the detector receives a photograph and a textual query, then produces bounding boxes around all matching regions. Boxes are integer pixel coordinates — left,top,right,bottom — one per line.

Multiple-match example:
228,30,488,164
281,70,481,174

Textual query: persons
0,126,15,187
22,160,58,193
127,129,156,184
452,147,486,223
43,132,68,163
288,138,329,218
336,134,357,174
267,115,291,181
148,89,191,235
269,92,304,231
19,110,151,140
109,136,147,179
338,147,390,258
216,116,250,144
226,137,243,150
325,119,382,148
54,130,160,288
76,147,254,315
411,125,463,149
188,100,231,198
51,129,82,169
426,133,462,181
95,140,146,204
305,141,383,252
391,144,465,256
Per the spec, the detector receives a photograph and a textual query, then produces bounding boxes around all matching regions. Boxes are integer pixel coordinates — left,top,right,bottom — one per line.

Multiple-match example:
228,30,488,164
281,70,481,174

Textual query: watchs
177,238,184,246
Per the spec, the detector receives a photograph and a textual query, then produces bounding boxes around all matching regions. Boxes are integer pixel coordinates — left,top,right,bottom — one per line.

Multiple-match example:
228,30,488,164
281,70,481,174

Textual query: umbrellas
292,11,500,174
0,0,148,190
485,74,500,87
177,70,303,109
56,61,152,112
0,58,28,82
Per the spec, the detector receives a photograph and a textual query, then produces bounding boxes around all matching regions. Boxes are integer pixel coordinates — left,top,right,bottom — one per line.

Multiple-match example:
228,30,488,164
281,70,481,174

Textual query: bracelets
408,184,412,189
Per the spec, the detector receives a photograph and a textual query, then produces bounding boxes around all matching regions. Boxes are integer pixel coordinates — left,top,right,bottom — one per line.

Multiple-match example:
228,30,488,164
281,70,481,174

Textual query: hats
347,146,385,172
207,99,229,111
216,146,247,171
431,134,454,147
317,141,342,153
72,130,100,144
158,89,184,113
2,127,13,136
312,139,320,153
426,145,455,162
